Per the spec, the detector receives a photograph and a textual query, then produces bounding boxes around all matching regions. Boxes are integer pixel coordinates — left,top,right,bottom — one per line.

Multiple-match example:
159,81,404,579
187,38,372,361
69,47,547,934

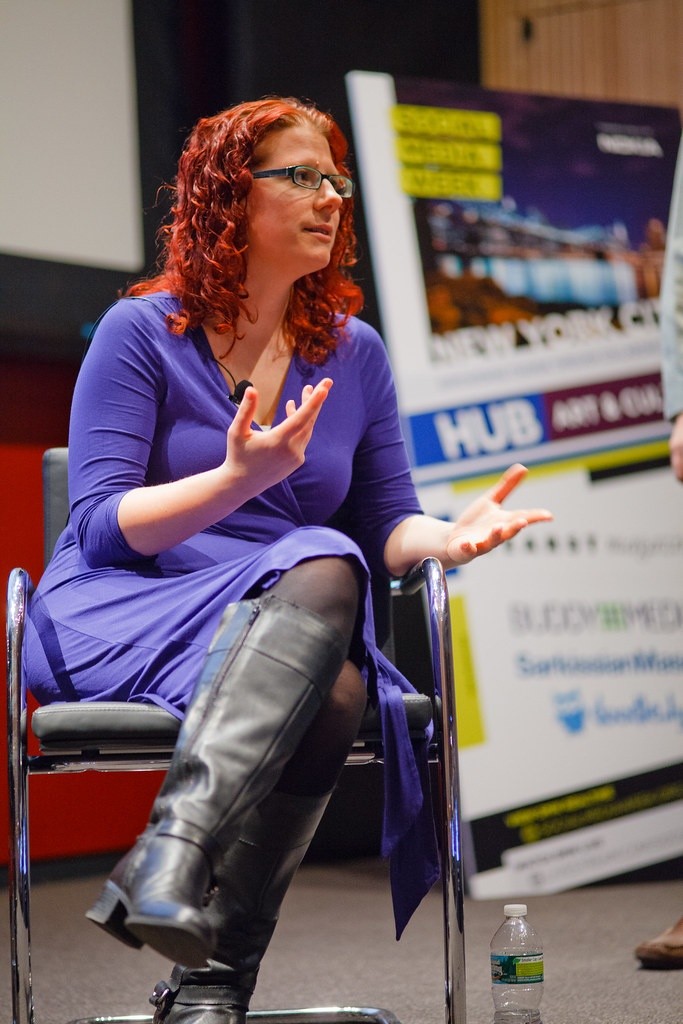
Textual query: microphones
228,380,253,404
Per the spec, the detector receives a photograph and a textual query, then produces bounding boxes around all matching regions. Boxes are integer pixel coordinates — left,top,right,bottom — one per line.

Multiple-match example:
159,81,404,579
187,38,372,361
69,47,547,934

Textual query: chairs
8,448,470,1022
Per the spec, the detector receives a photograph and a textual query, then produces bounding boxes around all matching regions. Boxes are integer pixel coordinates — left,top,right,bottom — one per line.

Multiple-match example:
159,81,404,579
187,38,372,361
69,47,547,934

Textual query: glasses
253,164,354,198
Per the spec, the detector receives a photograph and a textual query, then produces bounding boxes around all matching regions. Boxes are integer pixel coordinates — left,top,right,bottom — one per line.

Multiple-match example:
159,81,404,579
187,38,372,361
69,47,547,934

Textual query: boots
84,595,346,968
151,783,335,1024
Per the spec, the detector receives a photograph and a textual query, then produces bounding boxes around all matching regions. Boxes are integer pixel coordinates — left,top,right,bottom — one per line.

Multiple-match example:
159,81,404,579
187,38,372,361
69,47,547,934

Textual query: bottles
489,904,545,1024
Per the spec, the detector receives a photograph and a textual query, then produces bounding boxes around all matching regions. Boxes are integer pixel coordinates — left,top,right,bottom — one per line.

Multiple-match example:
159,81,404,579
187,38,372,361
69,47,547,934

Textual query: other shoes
635,915,683,964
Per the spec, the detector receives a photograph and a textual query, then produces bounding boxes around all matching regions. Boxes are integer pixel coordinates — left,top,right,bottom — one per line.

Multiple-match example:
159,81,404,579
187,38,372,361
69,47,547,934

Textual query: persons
637,144,683,967
21,101,554,1024
